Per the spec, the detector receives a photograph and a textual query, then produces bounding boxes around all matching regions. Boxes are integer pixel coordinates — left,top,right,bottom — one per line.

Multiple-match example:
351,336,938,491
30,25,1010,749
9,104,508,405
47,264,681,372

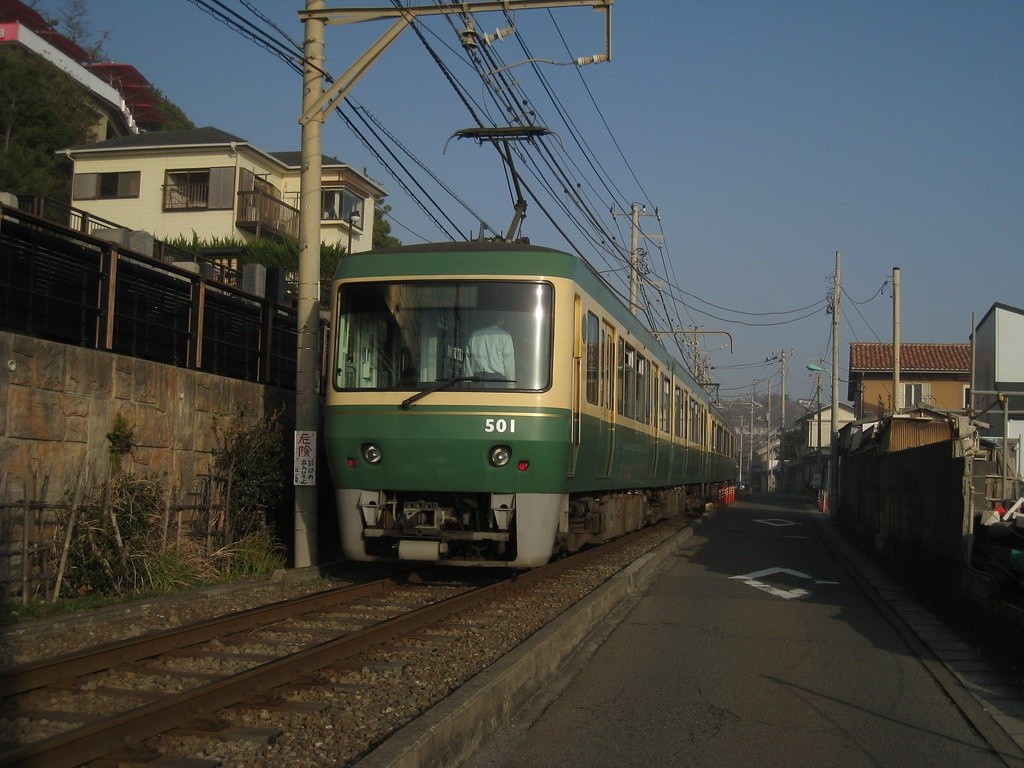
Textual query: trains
322,125,737,570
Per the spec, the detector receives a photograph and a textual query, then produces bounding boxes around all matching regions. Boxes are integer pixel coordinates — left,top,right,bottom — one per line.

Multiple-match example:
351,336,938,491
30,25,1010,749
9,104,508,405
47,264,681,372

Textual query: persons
464,311,517,387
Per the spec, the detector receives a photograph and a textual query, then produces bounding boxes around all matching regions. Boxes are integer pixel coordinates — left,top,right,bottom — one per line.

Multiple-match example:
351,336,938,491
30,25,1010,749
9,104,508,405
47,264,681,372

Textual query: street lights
805,363,840,524
